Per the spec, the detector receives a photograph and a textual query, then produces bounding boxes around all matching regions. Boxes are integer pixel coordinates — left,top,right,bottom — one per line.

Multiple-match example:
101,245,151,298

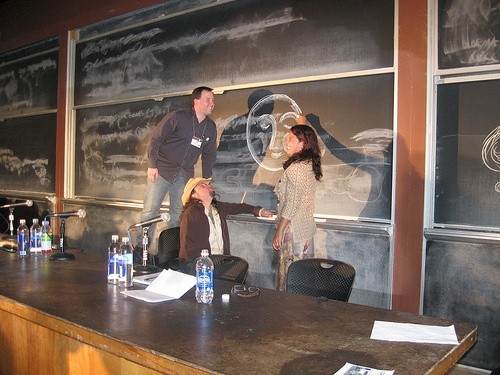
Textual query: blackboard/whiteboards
0,0,500,374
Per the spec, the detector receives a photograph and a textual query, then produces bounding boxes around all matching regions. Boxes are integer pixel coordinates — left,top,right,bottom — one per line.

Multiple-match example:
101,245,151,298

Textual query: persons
272,116,323,292
179,178,273,263
134,86,217,263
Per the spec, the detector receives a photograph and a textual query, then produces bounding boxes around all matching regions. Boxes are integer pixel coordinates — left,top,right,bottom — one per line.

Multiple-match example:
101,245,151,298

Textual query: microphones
0,199,33,208
47,208,86,218
129,212,170,228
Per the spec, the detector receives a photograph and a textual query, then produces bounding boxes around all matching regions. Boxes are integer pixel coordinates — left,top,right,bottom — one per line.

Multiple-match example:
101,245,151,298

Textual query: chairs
152,228,181,272
181,254,249,285
0,196,51,236
287,259,357,304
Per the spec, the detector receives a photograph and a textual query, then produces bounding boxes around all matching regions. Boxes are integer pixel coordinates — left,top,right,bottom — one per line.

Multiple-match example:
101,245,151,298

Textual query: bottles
107,235,122,284
30,219,42,253
40,220,52,253
195,249,214,304
17,219,29,258
117,237,134,288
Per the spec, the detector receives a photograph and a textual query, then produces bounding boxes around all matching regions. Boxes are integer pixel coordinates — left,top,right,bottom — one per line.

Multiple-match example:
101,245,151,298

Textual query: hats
181,177,212,207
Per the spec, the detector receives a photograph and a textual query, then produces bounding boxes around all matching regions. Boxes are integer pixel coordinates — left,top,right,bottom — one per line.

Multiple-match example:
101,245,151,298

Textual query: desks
0,248,479,375
